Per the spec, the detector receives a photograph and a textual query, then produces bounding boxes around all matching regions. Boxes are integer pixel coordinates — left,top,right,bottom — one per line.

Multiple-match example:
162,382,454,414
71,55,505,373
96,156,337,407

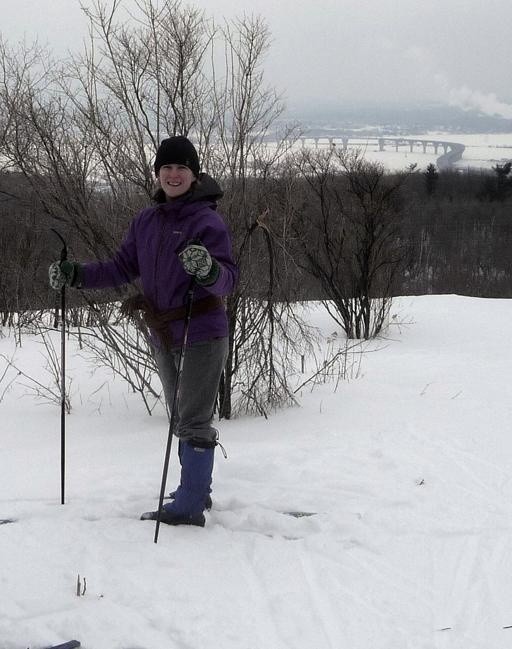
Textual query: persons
49,135,240,527
